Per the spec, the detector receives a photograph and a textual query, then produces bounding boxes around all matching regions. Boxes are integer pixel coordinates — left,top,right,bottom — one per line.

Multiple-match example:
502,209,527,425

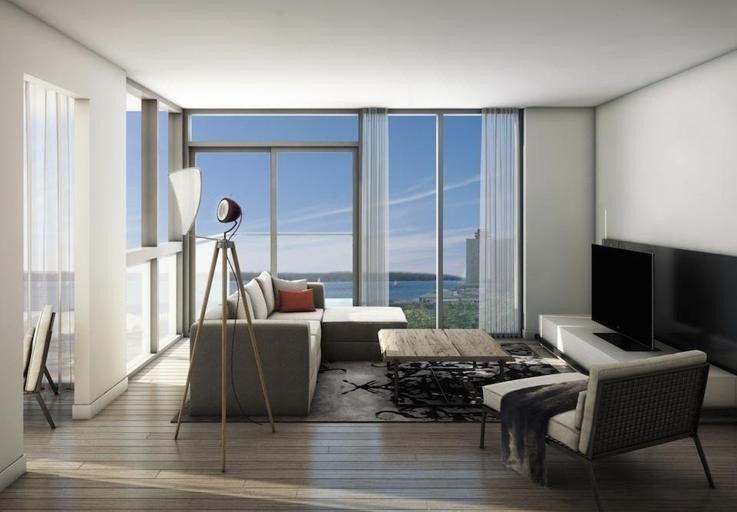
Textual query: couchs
189,282,407,416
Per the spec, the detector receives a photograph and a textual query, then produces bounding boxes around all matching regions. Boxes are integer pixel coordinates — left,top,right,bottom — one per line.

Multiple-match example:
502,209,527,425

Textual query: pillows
272,277,307,311
279,289,315,313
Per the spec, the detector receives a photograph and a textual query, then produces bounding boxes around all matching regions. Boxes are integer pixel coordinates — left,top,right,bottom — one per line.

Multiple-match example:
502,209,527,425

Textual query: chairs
23,304,59,430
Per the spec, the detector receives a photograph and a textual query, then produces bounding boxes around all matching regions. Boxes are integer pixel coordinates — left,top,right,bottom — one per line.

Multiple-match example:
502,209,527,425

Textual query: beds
479,350,714,511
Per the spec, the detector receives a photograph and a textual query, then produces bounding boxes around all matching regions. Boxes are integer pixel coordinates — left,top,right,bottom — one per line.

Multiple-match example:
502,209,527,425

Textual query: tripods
174,238,278,474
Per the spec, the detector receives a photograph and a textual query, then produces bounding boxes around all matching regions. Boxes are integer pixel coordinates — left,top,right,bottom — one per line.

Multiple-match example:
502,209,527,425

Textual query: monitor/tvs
590,244,663,352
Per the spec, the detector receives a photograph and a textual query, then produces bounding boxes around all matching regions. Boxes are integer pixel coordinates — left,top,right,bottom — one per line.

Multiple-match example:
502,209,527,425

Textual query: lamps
216,199,242,239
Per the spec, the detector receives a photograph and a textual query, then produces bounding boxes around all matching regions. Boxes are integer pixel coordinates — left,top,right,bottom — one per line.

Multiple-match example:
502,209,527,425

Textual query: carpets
171,339,577,423
